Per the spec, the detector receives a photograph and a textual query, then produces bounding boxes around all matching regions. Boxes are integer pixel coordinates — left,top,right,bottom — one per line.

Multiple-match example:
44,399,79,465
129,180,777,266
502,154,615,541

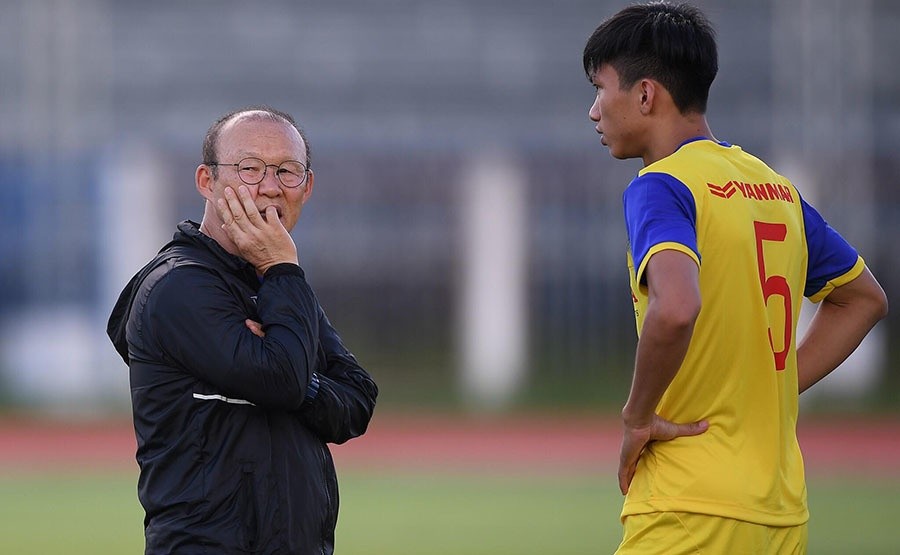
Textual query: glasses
207,157,309,188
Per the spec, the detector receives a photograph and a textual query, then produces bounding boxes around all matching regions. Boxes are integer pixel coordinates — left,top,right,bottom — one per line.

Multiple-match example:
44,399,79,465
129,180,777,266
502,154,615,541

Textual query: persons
582,1,889,555
125,106,378,555
